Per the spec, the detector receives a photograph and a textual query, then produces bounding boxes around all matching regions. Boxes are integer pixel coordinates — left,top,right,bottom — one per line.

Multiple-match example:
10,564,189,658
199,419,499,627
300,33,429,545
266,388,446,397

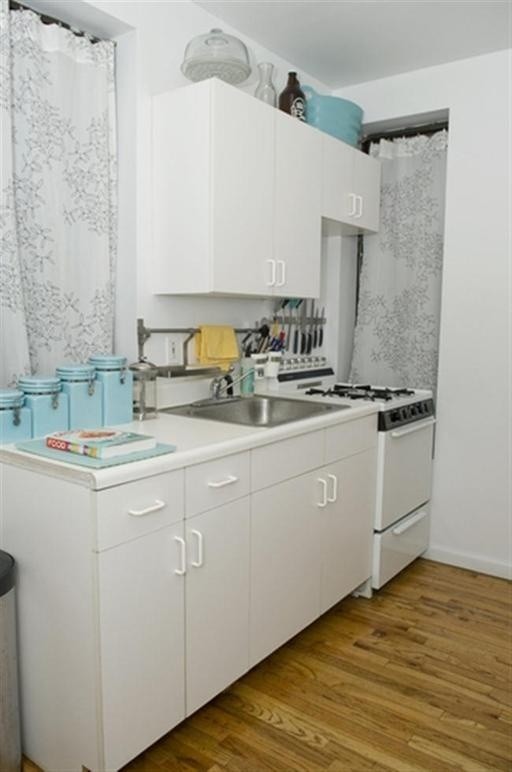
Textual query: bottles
279,70,307,123
254,63,279,106
233,345,258,399
0,354,134,443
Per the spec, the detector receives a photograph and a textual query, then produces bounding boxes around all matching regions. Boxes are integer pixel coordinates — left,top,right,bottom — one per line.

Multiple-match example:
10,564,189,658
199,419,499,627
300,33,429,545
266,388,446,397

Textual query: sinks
158,394,352,430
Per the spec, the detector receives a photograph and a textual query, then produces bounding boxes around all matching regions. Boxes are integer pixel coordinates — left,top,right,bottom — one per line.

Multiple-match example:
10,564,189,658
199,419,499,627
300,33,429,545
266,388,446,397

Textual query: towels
195,324,240,373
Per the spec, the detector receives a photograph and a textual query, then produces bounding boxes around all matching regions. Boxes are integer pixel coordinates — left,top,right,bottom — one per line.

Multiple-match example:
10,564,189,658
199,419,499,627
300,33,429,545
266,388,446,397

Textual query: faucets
211,367,257,399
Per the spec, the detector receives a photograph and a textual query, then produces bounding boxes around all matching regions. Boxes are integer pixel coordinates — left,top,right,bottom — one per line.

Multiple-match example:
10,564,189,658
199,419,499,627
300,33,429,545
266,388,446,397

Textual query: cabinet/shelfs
250,411,378,670
152,76,322,300
321,131,381,235
0,450,250,772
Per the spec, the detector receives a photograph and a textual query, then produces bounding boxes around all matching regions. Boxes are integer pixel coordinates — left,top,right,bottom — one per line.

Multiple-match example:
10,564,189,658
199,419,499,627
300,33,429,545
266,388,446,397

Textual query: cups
251,350,281,380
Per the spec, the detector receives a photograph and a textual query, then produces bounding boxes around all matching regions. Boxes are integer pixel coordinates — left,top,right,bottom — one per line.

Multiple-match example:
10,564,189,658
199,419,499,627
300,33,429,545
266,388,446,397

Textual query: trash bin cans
0,548,22,772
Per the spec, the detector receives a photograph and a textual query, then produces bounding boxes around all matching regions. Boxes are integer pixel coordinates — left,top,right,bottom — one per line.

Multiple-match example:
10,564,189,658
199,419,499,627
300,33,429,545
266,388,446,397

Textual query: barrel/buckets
299,84,365,147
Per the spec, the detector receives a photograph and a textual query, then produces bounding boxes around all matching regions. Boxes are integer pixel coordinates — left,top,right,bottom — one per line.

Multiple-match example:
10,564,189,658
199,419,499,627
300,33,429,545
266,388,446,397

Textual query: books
46,428,157,458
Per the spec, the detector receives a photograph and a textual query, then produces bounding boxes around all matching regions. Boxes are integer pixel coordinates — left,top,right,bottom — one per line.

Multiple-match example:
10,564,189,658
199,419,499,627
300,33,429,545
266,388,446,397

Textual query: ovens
377,416,435,535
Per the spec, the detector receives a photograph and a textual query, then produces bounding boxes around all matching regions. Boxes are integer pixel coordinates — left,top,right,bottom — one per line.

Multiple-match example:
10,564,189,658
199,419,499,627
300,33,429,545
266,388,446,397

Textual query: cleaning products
240,340,254,399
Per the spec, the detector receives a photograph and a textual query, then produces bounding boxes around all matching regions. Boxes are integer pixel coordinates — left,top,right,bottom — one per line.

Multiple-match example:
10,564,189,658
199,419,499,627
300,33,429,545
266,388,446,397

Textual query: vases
254,62,277,108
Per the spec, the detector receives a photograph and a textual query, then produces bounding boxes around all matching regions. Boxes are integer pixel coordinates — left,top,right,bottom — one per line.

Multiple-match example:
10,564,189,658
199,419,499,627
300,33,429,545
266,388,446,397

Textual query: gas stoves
305,385,437,429
